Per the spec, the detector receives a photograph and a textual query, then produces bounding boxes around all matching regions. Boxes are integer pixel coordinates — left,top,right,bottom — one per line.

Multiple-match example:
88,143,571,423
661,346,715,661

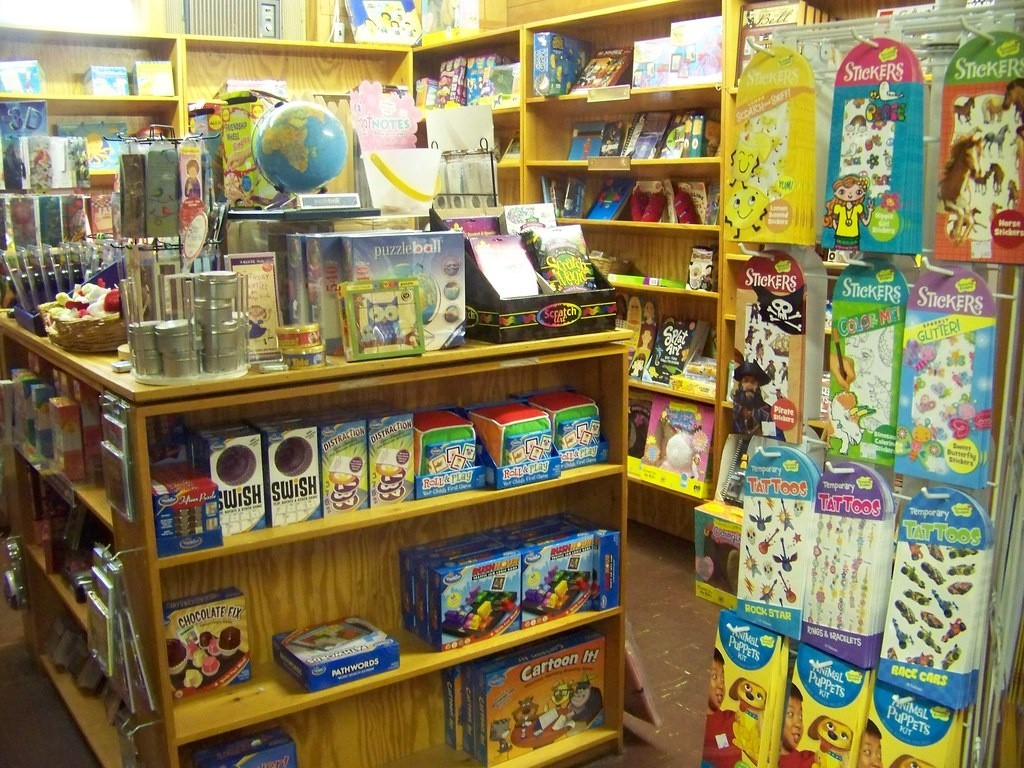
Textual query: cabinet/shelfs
126,339,629,768
0,315,141,768
521,0,729,512
408,24,522,206
181,35,413,151
0,29,187,198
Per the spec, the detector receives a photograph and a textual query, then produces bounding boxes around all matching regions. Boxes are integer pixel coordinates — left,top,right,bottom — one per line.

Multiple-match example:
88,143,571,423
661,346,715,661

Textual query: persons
777,683,816,768
700,645,742,768
855,717,883,768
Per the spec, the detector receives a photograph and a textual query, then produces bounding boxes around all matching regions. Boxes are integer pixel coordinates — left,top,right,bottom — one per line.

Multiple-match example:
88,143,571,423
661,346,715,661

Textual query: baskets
37,300,128,352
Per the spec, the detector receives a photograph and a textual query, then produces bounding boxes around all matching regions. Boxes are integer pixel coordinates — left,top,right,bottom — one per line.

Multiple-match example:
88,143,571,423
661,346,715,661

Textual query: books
568,109,711,163
500,128,521,164
541,173,720,227
721,438,752,508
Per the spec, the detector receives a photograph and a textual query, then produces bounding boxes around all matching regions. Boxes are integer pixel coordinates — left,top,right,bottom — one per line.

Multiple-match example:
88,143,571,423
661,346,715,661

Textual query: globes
251,100,349,212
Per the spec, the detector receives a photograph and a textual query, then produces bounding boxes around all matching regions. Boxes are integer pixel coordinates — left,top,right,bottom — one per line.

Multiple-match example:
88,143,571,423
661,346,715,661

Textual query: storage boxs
399,509,622,653
283,205,618,363
689,114,721,158
531,15,723,97
146,384,611,558
272,615,401,693
734,0,936,87
0,60,49,96
13,256,126,337
85,64,130,98
162,586,252,702
414,51,521,110
189,726,297,768
438,625,606,768
693,501,744,612
12,349,106,484
0,100,130,189
131,60,175,97
687,245,718,292
344,0,422,48
420,0,481,47
628,386,717,499
